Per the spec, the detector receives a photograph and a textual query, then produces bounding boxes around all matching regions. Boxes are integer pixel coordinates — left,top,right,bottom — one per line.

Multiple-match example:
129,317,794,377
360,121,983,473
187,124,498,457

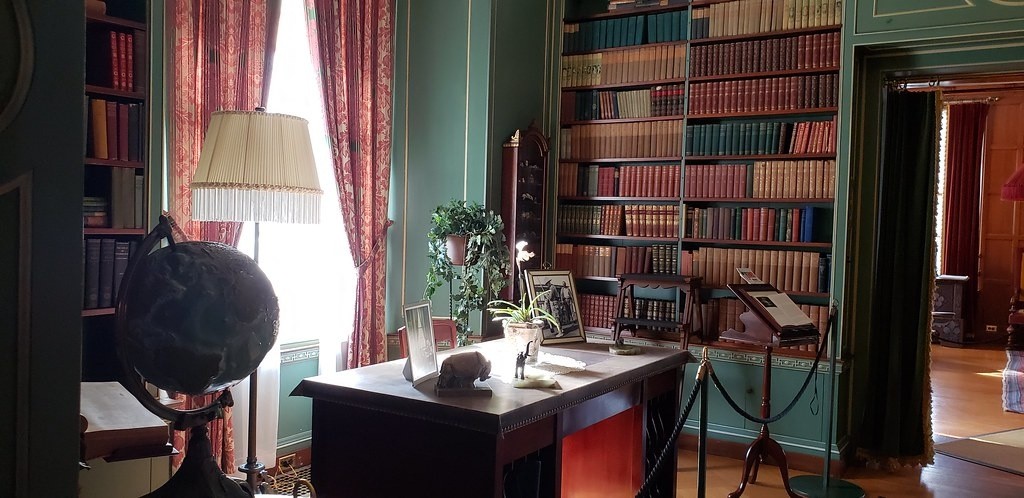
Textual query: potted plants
487,290,561,357
422,198,512,346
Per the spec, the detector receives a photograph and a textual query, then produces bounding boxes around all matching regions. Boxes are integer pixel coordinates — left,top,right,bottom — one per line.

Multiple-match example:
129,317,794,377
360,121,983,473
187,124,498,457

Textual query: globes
112,214,280,498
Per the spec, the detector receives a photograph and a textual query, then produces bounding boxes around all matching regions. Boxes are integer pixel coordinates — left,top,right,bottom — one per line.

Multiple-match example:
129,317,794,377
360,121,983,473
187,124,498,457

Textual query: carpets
1002,350,1024,414
933,427,1024,476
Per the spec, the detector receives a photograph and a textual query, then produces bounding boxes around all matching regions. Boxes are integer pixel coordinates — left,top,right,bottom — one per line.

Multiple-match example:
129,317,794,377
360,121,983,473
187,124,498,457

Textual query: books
76,0,149,312
540,0,846,361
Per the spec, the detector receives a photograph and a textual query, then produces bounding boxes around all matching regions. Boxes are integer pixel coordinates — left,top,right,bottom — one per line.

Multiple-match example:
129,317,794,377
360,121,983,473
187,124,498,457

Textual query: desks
288,337,699,498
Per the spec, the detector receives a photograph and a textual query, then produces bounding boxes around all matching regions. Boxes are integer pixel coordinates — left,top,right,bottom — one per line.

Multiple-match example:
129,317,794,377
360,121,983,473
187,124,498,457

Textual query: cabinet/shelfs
78,0,179,498
502,119,552,301
932,275,970,348
552,0,854,477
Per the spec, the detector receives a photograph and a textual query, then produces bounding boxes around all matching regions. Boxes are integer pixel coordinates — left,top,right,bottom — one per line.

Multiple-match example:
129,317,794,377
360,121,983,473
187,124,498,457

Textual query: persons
542,277,565,337
558,279,577,326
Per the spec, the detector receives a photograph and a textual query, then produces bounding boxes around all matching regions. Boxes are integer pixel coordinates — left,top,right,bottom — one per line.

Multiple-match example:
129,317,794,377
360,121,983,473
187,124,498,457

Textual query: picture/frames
402,301,439,388
524,269,586,346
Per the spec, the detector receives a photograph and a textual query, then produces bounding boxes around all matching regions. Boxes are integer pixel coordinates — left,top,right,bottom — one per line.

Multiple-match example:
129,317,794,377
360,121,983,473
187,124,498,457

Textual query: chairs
398,318,456,359
1006,247,1024,350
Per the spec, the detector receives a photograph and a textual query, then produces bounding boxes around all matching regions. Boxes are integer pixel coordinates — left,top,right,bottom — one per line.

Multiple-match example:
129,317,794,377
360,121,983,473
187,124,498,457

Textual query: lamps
190,106,324,494
